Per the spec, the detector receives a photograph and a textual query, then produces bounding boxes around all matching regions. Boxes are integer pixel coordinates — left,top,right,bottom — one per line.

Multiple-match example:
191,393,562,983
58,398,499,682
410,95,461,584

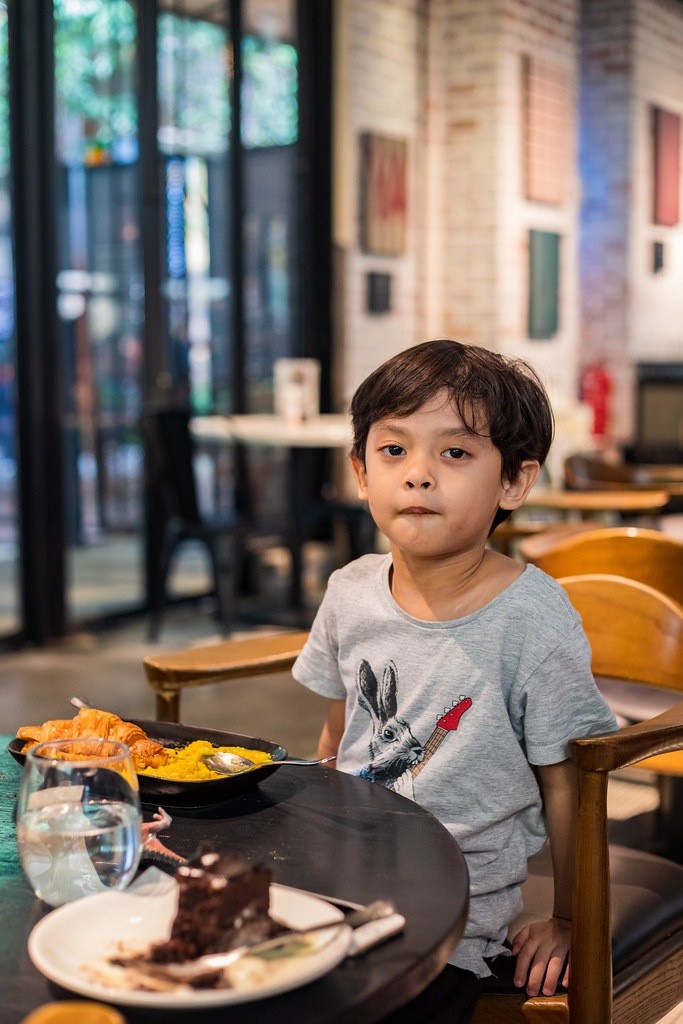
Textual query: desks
186,406,362,633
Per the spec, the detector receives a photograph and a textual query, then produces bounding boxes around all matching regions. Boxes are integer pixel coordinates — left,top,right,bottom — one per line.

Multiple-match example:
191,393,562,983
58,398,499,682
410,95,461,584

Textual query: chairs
491,491,670,553
128,402,273,646
518,526,683,614
136,572,683,1024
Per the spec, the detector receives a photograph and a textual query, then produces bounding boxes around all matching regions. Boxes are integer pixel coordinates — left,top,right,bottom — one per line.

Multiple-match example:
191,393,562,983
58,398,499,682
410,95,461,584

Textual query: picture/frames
524,52,567,206
652,101,682,226
358,131,410,256
527,229,564,336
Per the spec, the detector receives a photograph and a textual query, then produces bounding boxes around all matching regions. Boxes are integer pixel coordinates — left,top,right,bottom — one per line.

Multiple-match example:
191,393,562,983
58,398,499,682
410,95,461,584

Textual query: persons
287,337,621,1024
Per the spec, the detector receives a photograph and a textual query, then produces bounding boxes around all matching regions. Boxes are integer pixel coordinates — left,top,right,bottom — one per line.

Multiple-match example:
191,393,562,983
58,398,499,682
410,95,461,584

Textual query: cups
273,354,320,425
19,732,142,908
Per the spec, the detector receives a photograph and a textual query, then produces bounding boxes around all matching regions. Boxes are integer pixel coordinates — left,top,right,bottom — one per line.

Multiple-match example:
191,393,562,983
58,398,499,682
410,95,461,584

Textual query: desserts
156,850,299,987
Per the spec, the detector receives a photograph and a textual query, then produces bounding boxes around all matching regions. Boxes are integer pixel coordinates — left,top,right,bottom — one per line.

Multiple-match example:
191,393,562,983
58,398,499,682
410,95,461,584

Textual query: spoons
132,897,396,979
200,752,338,775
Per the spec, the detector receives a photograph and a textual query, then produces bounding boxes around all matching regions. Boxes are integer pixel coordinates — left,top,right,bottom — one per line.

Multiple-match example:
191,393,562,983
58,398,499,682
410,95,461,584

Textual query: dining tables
0,709,472,1024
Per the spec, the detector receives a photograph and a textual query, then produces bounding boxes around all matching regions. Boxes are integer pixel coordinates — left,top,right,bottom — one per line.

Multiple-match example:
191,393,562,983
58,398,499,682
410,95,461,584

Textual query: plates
28,868,354,1007
9,716,287,806
